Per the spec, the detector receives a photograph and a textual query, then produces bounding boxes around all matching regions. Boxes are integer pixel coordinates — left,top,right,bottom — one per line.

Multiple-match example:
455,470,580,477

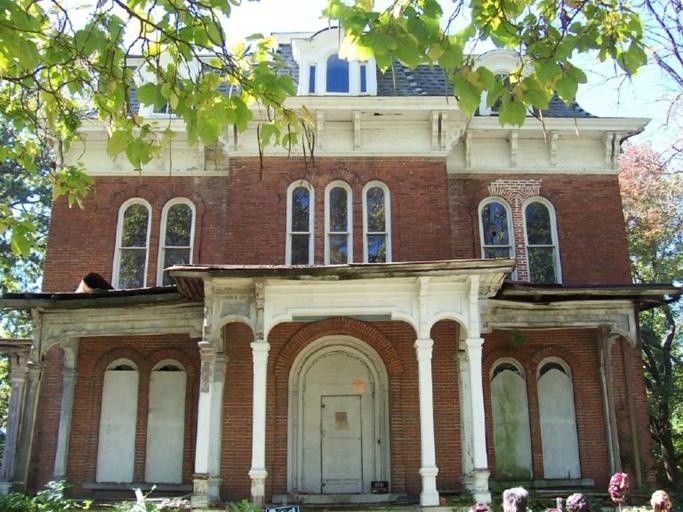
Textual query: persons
650,490,672,512
566,492,590,512
502,486,529,511
